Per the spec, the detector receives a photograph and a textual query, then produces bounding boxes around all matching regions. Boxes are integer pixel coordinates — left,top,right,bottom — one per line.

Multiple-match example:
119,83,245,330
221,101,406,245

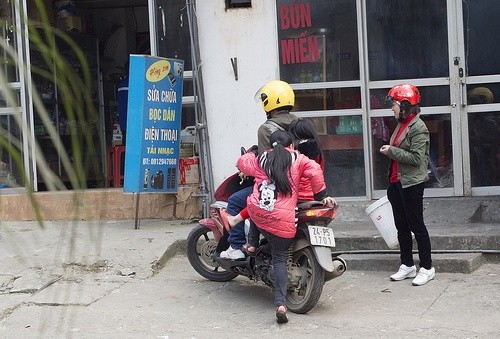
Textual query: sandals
241,244,257,256
275,305,289,323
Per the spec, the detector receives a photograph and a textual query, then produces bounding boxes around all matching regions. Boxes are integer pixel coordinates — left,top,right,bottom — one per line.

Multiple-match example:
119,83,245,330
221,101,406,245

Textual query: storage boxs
178,157,199,185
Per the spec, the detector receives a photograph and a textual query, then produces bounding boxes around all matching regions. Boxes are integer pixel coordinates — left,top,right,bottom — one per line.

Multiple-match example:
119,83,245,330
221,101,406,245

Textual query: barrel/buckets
365,195,415,250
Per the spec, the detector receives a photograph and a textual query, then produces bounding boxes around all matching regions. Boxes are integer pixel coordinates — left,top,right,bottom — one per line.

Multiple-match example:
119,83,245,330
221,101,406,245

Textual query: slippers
219,207,233,232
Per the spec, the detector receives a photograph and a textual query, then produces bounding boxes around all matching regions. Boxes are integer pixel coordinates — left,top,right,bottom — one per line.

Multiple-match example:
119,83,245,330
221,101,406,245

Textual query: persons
219,81,338,325
380,84,436,286
467,87,500,190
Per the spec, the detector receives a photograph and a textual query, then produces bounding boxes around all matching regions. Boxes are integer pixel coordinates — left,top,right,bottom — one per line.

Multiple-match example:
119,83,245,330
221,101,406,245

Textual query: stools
105,146,125,188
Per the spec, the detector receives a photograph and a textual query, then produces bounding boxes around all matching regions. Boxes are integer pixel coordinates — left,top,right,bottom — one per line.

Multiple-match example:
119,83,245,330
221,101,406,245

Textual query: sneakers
389,264,417,281
219,245,246,261
412,267,436,286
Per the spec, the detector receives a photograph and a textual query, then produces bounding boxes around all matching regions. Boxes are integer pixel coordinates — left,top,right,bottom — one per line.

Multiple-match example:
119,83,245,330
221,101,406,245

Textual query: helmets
387,83,422,106
471,87,494,104
260,79,295,113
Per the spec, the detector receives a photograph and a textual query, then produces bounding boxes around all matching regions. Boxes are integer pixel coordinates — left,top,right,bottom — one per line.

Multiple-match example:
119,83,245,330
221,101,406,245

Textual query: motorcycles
184,145,347,315
